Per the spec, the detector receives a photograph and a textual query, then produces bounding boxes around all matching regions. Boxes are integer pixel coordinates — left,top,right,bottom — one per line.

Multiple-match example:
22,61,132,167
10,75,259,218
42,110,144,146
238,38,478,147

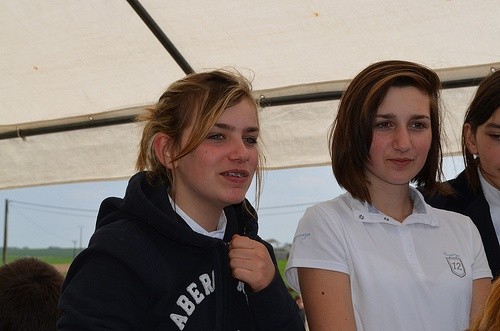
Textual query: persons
284,60,500,331
57,71,305,331
0,258,65,331
